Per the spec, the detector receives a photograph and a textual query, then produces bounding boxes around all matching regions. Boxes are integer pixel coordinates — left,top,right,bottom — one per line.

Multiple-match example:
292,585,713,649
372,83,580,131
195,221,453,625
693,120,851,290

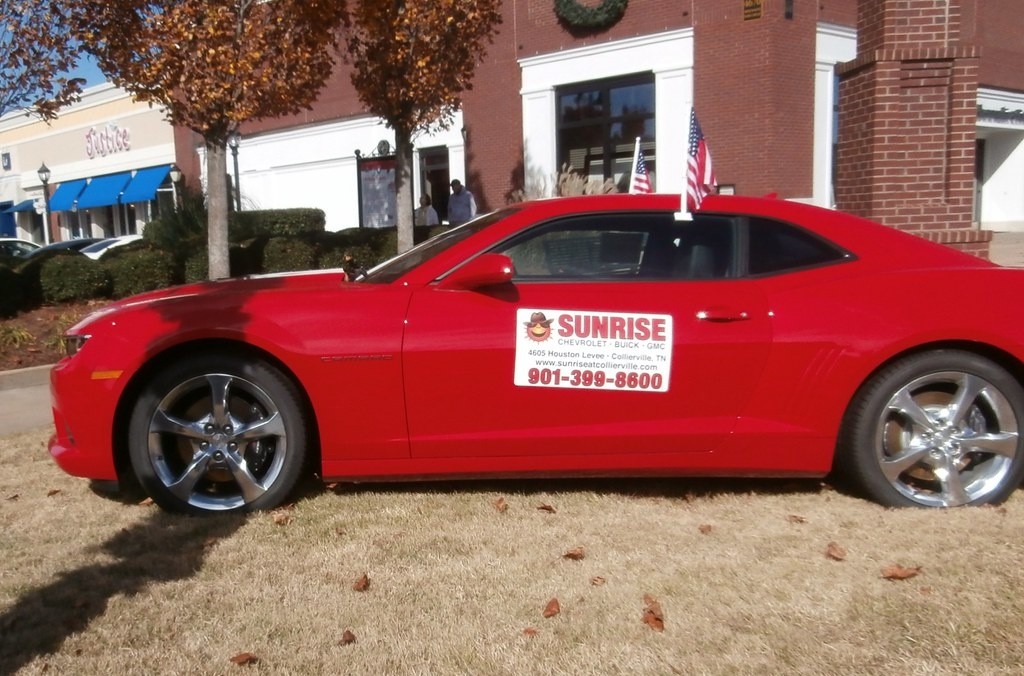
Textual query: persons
414,194,438,225
448,179,477,222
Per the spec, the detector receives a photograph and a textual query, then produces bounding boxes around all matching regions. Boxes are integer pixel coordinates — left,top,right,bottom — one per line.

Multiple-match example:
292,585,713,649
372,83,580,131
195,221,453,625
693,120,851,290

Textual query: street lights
38,160,51,250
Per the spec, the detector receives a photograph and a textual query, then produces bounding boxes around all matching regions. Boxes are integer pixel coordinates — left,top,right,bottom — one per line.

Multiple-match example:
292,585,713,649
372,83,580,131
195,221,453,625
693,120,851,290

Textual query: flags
631,144,651,194
687,108,717,209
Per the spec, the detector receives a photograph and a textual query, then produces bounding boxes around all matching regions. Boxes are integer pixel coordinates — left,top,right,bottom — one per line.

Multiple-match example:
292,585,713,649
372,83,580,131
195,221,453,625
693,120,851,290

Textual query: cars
80,235,143,263
49,192,1024,516
24,238,108,256
0,237,43,261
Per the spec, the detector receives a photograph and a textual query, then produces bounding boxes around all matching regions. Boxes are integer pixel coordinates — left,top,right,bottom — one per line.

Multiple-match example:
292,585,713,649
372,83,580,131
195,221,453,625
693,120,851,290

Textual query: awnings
49,178,86,211
2,198,39,212
76,168,131,209
120,163,170,203
